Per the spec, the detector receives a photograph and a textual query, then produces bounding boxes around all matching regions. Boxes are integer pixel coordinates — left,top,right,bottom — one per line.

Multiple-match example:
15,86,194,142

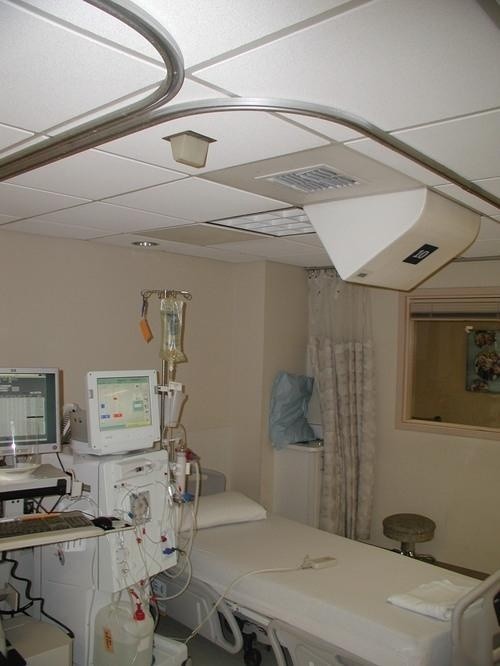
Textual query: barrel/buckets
93,601,155,666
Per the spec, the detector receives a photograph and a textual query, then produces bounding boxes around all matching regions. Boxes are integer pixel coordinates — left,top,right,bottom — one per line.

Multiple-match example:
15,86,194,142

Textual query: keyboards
0,510,94,541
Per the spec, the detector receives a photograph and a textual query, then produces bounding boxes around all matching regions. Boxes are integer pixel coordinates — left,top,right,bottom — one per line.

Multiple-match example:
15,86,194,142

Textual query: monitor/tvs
85,369,163,456
0,367,62,473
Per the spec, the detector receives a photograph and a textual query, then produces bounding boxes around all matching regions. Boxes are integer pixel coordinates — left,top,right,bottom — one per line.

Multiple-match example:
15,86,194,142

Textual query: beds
150,465,500,666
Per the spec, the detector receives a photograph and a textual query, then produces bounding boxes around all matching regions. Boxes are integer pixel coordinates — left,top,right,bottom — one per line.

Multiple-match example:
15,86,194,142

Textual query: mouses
92,516,113,530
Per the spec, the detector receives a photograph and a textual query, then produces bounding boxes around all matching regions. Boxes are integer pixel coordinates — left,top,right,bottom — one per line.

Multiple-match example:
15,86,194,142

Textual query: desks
271,437,324,531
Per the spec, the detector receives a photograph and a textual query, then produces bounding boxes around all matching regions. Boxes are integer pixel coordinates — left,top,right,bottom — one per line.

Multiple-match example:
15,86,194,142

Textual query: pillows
267,371,316,449
177,490,267,533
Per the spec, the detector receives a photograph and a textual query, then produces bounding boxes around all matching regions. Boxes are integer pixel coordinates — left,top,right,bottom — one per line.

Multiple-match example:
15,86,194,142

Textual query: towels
386,579,483,622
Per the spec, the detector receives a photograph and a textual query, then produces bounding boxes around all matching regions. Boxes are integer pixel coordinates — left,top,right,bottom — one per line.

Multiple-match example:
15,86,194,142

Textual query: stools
382,513,436,563
